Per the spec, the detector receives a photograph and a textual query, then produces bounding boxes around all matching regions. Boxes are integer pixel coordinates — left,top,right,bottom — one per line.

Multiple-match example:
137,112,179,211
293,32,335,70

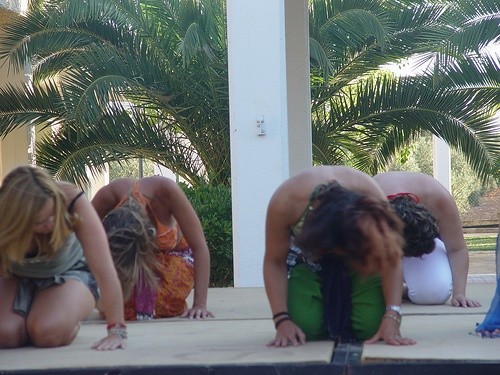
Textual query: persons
263,165,417,348
90,176,216,321
0,165,130,352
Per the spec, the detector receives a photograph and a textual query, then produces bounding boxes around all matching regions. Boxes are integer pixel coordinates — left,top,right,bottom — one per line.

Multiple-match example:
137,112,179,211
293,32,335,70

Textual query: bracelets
273,311,289,319
385,305,402,317
384,314,402,326
105,323,127,339
274,316,292,329
371,171,483,309
